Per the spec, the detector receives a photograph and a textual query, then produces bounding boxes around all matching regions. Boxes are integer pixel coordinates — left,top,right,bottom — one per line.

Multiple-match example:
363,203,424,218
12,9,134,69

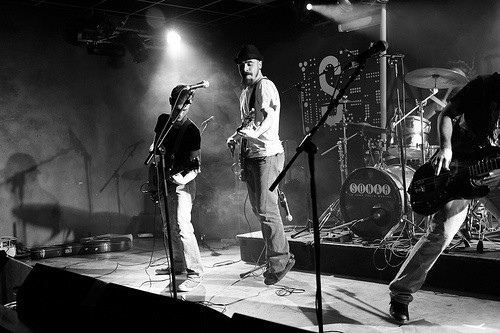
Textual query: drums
387,116,431,160
338,164,425,239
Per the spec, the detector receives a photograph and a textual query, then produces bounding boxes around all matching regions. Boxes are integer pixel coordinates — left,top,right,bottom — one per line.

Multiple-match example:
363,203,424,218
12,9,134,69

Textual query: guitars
409,156,500,215
148,154,201,201
237,106,256,181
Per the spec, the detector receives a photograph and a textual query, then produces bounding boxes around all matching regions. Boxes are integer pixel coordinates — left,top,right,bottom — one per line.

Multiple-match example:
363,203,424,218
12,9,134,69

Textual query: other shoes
155,267,182,275
264,259,295,285
390,299,410,325
263,253,294,277
177,274,208,291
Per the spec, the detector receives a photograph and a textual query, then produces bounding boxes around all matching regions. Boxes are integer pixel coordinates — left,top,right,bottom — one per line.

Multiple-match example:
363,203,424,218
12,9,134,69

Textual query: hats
234,44,264,64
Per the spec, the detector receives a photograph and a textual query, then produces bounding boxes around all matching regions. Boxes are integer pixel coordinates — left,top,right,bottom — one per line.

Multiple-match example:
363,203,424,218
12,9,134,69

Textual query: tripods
377,76,500,251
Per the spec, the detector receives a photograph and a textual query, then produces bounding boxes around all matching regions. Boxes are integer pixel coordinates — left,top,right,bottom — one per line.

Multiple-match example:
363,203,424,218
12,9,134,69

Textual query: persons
388,71,500,322
149,85,204,293
226,45,295,285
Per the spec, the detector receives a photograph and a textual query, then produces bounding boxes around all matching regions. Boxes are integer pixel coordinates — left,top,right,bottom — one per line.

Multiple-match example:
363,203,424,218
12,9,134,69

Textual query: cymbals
320,99,371,105
335,122,386,134
405,68,467,89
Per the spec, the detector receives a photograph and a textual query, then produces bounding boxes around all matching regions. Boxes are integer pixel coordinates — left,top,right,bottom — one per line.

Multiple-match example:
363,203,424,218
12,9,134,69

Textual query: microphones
282,192,293,221
372,212,381,219
183,80,209,91
201,116,215,126
341,40,388,71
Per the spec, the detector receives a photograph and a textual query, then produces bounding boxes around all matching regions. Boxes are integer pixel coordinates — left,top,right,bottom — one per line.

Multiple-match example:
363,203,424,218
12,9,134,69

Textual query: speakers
16,262,318,333
